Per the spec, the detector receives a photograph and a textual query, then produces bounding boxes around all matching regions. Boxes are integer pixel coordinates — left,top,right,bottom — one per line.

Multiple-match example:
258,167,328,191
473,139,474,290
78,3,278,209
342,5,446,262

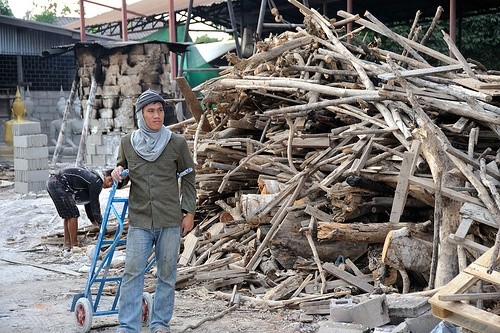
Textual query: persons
46,168,124,251
111,90,196,333
4,85,85,155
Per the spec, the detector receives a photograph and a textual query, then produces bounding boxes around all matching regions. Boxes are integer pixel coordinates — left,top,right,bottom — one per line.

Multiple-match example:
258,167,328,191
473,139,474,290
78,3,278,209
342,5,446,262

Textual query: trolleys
68,168,194,333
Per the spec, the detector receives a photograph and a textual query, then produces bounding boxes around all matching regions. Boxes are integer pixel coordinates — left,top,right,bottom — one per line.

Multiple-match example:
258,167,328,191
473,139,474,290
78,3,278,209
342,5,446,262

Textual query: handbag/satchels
74,190,91,204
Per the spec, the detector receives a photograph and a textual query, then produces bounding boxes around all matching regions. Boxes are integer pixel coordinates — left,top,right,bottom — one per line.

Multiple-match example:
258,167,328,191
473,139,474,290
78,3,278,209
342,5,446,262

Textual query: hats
135,92,166,112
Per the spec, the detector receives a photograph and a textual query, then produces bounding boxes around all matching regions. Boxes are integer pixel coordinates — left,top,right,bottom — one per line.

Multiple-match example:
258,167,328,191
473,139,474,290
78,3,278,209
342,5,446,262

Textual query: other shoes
153,328,171,333
114,330,125,333
61,246,82,258
